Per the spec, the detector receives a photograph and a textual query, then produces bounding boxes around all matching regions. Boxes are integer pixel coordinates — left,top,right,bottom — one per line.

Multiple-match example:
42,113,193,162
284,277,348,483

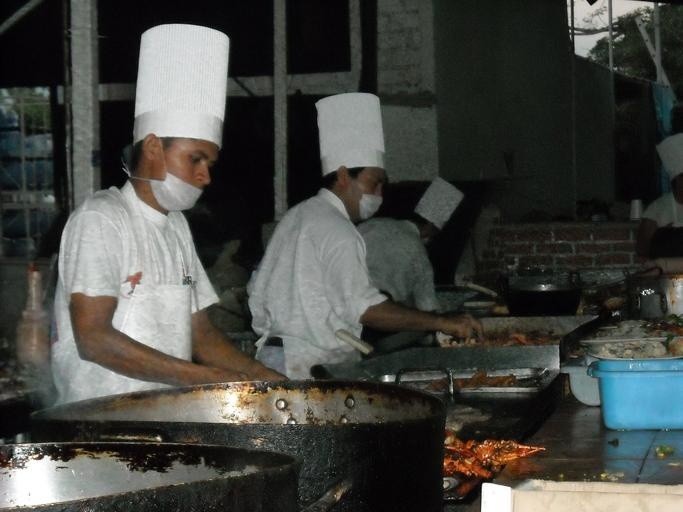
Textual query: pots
465,279,585,314
620,264,682,319
0,361,454,512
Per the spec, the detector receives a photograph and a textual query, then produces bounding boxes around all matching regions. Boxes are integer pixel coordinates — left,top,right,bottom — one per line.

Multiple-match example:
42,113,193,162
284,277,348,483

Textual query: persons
354,211,444,317
44,131,290,409
634,172,682,258
243,164,484,381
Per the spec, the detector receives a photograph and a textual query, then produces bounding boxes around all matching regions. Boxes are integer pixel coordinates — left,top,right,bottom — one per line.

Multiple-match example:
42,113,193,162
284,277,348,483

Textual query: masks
348,180,383,222
141,155,203,212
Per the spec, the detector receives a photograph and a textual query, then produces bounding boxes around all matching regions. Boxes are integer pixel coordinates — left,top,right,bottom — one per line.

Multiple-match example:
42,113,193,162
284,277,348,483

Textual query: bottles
14,268,50,362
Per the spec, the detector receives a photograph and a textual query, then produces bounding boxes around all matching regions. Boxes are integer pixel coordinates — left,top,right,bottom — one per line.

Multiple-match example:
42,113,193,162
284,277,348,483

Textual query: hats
315,92,386,176
133,23,230,151
654,133,683,179
415,177,464,229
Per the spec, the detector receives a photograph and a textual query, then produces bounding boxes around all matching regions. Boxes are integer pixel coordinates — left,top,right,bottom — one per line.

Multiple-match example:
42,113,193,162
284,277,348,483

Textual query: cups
629,198,643,221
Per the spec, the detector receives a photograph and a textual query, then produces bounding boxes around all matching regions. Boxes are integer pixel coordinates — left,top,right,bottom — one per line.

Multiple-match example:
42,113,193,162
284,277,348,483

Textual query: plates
578,323,683,359
